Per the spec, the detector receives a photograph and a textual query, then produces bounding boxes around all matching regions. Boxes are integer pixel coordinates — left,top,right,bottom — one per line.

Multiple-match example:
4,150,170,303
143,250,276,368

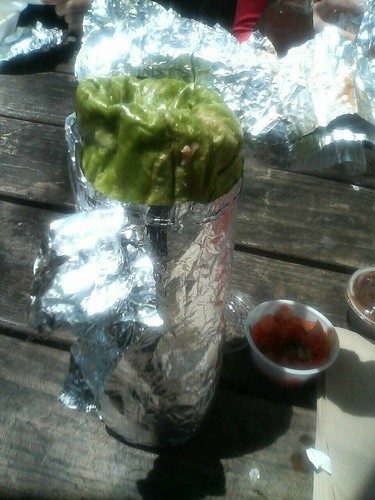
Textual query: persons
39,1,273,56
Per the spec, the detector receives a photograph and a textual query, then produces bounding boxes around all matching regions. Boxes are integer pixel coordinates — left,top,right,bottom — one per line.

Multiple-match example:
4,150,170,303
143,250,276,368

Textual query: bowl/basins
344,267,375,338
245,299,340,391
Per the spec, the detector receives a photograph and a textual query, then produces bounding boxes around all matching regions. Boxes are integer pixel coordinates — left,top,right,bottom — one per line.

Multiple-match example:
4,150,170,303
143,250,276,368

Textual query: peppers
72,75,245,205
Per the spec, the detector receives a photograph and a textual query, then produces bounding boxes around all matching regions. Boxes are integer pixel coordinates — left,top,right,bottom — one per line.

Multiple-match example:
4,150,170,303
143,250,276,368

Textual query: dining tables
0,70,374,500
